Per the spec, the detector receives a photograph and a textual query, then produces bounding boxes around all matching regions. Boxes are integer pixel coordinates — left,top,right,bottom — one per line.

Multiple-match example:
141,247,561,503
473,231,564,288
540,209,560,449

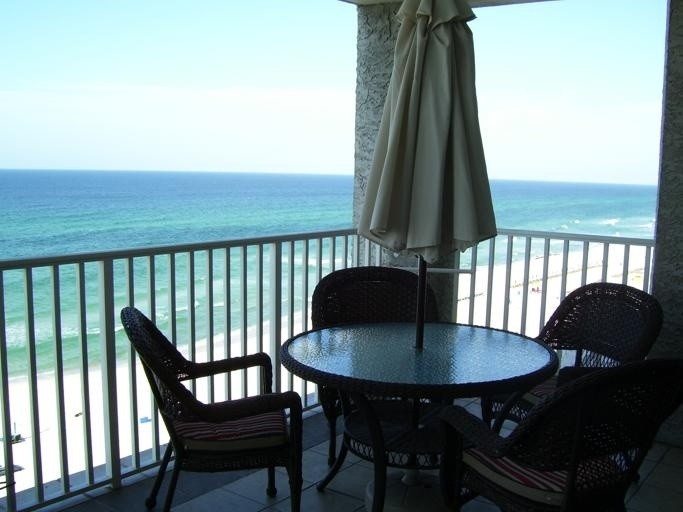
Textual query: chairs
118,307,303,511
310,263,437,468
479,280,665,432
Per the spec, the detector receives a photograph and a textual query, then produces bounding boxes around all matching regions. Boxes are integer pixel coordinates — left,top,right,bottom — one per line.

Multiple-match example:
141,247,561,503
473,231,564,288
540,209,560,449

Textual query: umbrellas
356,1,498,350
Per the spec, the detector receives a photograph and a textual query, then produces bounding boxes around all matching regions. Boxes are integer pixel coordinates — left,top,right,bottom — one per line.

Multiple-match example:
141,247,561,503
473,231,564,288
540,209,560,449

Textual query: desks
278,320,557,512
437,352,681,512
